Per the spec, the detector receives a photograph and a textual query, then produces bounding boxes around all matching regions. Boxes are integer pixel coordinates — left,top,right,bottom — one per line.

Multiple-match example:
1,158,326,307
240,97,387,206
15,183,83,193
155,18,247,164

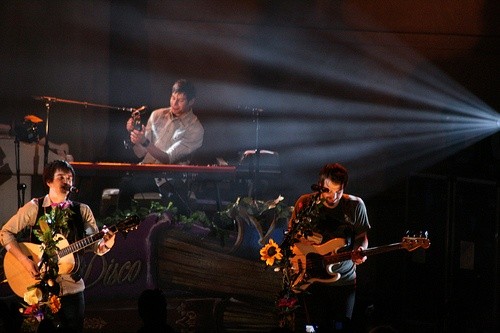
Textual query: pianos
68,160,237,180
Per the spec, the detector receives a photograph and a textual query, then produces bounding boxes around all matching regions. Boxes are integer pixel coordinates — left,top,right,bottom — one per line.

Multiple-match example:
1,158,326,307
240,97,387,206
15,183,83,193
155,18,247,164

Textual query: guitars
287,230,431,294
3,216,141,297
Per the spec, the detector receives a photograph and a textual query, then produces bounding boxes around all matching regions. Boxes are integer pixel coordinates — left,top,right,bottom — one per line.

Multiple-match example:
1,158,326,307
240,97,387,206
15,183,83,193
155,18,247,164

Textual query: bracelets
142,140,150,148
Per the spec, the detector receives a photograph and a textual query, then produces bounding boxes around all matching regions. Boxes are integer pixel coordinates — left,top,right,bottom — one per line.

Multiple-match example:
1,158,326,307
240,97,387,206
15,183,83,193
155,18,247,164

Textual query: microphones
311,184,329,193
132,106,148,116
65,185,78,193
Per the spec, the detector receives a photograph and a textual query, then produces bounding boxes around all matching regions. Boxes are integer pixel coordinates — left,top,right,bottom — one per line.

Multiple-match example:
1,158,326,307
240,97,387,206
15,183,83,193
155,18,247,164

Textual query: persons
1,160,116,333
281,162,371,333
118,79,205,213
